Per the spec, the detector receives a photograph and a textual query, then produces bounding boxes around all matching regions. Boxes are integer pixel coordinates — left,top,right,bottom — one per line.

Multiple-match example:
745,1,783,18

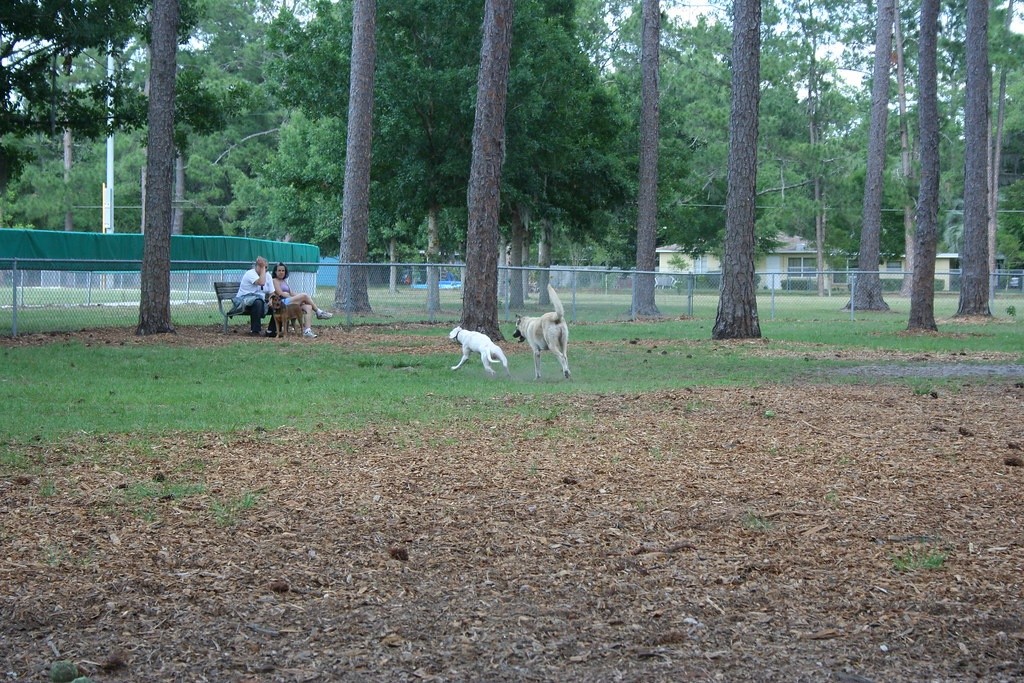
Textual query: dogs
270,294,307,340
512,284,572,381
449,325,510,376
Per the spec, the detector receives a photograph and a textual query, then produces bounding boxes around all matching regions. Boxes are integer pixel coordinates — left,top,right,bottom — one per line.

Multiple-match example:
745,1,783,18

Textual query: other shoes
251,330,261,335
266,331,276,337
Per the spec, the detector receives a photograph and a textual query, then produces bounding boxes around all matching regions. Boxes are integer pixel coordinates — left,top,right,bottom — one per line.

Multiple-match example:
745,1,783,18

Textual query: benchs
214,282,273,334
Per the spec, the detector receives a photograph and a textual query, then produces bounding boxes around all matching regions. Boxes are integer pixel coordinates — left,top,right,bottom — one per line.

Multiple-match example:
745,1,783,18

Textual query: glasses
278,270,284,271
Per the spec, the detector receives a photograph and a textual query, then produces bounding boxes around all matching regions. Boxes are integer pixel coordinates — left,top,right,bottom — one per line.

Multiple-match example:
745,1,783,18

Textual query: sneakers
303,330,318,339
317,311,333,319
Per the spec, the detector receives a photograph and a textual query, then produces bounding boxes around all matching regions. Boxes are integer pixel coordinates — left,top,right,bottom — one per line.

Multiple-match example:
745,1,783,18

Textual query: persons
237,257,284,339
272,262,333,338
